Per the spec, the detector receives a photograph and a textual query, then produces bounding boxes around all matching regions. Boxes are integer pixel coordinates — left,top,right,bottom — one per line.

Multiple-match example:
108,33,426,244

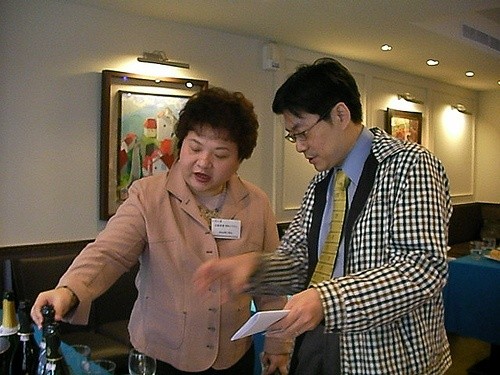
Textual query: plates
484,254,500,261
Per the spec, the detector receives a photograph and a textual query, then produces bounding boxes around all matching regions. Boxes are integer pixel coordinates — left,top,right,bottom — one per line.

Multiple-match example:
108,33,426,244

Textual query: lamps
452,103,472,115
397,91,424,105
137,50,191,69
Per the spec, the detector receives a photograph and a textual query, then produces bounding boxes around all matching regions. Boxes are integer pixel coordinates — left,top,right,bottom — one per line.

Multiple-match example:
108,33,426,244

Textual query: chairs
5,253,133,361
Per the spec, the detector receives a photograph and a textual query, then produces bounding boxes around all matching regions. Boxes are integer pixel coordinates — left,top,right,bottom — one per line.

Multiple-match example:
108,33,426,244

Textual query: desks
442,245,500,343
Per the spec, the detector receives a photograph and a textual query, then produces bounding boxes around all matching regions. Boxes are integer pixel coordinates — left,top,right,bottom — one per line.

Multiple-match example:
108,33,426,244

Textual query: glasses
285,117,323,143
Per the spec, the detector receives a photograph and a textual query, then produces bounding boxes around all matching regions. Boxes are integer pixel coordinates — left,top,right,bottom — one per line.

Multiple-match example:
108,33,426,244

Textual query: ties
307,170,351,289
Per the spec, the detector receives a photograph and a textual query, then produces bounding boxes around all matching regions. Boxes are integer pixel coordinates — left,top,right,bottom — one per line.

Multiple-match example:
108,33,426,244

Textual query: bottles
10,301,40,375
0,289,20,375
37,305,70,375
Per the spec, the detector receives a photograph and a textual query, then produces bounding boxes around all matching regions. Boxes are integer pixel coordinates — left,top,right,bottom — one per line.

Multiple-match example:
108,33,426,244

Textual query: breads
491,249,500,260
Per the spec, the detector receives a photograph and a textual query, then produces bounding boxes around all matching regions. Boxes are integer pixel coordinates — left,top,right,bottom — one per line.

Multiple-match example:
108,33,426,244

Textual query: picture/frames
387,106,422,147
100,68,209,222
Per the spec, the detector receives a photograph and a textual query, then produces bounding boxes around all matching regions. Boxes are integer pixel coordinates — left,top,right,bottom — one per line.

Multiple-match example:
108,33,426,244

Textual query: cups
80,359,116,375
60,345,91,375
469,231,496,255
129,348,156,375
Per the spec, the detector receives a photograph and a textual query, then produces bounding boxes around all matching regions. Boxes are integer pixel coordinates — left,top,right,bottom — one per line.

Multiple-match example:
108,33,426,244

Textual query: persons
191,58,453,375
29,88,292,375
